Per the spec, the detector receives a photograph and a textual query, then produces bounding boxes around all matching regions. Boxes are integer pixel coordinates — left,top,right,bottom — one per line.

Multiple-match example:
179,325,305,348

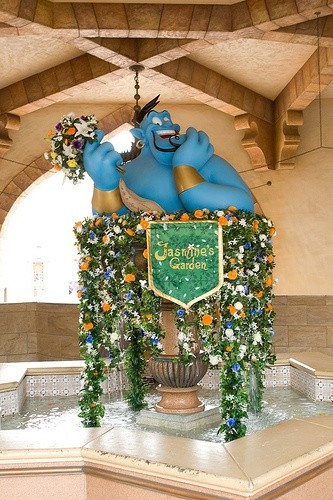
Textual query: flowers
43,112,126,186
74,209,280,444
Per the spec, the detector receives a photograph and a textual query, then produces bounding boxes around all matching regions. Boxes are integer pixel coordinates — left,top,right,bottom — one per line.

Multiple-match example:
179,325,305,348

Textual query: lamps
118,65,146,164
